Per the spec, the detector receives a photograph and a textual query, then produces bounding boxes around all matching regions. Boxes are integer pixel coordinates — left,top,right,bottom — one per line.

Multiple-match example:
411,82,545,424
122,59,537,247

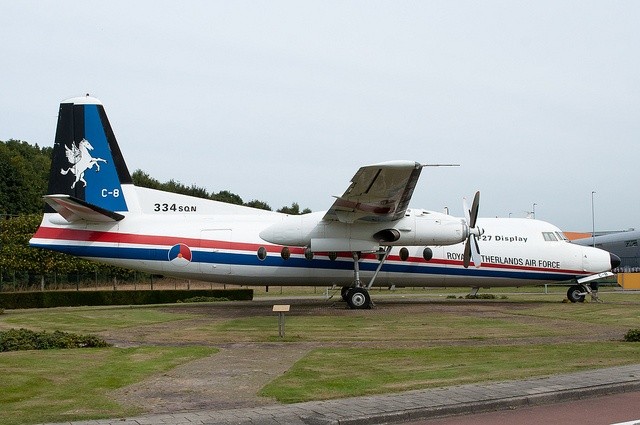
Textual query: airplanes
30,103,633,309
